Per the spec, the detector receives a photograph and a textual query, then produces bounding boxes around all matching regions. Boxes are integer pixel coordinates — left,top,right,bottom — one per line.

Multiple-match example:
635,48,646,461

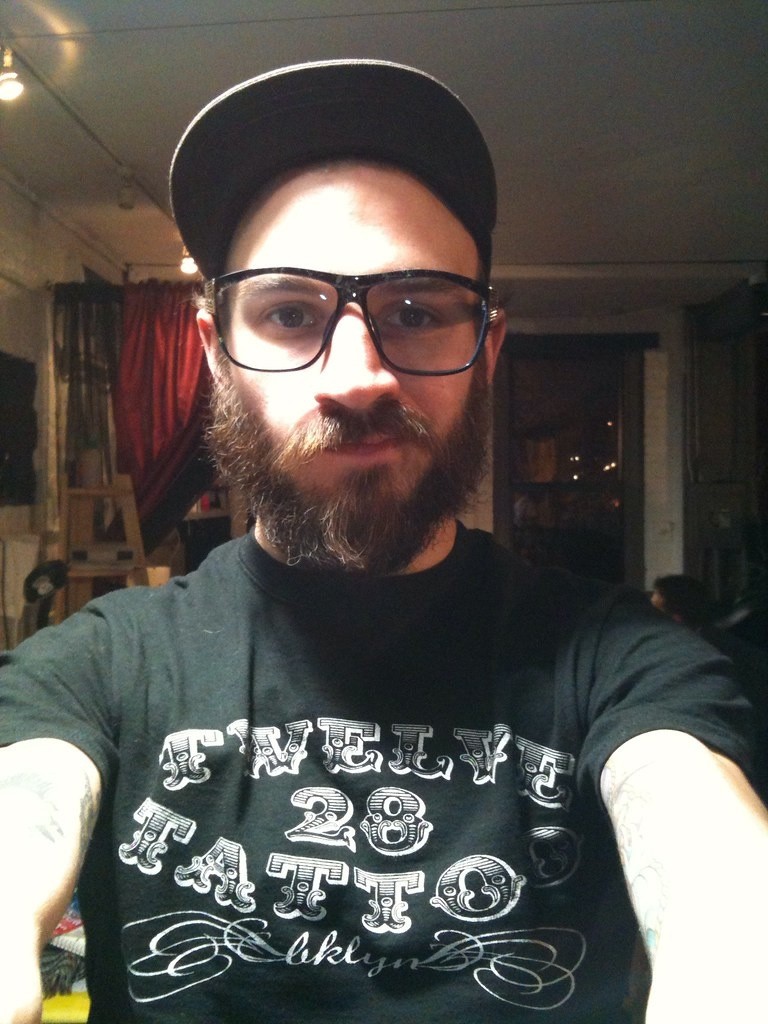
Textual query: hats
169,58,498,280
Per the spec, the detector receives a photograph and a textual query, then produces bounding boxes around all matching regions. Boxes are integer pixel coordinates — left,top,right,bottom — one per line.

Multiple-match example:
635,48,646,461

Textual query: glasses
204,267,499,376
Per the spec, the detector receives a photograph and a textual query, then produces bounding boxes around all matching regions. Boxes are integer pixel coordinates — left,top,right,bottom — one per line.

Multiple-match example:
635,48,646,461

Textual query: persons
646,573,768,714
0,57,768,1024
